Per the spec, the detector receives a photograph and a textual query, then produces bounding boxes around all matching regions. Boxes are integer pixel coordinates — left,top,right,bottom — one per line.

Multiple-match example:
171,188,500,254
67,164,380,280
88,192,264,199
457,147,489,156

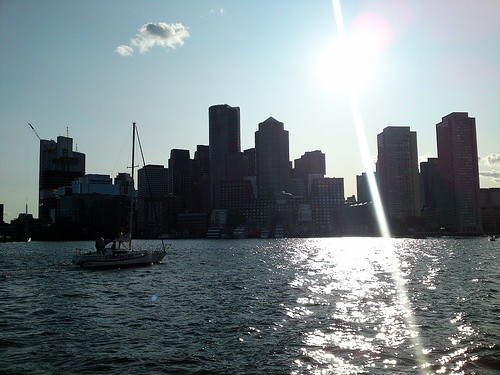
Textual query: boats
72,122,167,269
